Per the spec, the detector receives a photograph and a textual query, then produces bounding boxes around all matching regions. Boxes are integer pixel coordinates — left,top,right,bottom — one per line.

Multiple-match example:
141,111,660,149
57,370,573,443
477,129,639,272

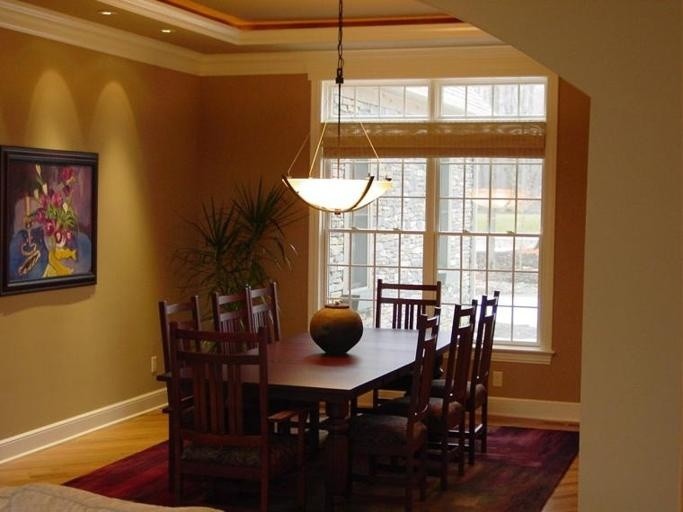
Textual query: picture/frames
0,144,99,299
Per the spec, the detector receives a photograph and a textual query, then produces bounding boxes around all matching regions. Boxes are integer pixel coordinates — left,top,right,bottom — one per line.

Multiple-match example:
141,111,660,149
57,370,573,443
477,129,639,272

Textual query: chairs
156,278,502,512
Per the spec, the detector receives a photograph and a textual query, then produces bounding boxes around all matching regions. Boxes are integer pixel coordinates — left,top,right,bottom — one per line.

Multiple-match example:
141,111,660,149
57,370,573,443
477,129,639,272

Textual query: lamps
278,0,397,212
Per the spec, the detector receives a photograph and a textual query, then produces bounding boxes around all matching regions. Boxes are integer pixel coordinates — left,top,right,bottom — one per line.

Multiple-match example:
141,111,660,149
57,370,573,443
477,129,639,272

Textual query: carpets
59,412,580,512
